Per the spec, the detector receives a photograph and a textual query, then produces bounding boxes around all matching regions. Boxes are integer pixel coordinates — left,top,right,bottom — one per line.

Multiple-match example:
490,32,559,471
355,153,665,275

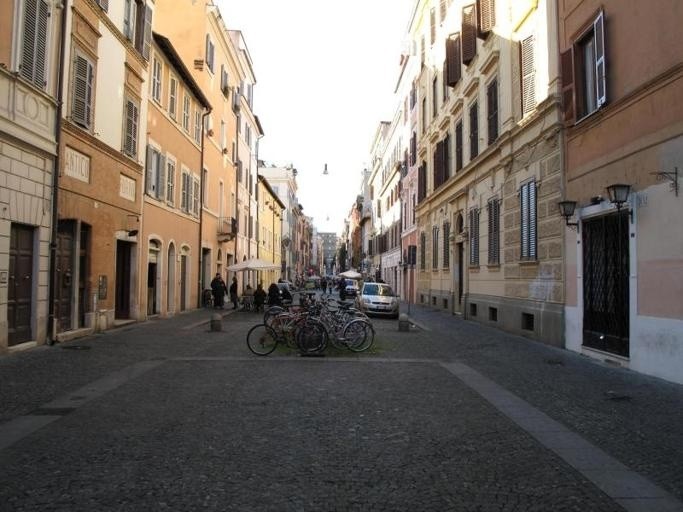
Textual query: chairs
238,295,284,312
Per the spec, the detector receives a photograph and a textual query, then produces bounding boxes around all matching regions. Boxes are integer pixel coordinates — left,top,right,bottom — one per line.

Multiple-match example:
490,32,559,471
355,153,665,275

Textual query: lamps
605,182,634,217
558,199,580,227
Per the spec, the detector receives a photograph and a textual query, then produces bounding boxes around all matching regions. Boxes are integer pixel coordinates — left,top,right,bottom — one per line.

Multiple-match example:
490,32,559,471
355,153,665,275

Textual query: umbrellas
338,269,362,279
308,274,321,280
223,257,283,288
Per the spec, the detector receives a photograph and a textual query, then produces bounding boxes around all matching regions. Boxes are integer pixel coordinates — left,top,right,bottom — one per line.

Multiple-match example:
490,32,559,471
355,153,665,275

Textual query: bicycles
245,289,376,357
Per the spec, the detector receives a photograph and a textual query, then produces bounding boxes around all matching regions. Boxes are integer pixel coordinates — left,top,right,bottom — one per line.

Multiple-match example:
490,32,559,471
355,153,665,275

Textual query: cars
276,282,294,298
354,281,400,319
304,279,315,290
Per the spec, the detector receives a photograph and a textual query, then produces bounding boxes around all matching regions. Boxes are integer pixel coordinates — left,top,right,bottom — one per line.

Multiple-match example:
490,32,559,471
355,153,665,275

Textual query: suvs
344,278,358,298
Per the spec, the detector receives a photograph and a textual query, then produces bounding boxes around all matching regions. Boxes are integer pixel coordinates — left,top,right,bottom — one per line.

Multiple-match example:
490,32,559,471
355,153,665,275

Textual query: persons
336,277,347,301
318,276,333,295
210,272,292,313
